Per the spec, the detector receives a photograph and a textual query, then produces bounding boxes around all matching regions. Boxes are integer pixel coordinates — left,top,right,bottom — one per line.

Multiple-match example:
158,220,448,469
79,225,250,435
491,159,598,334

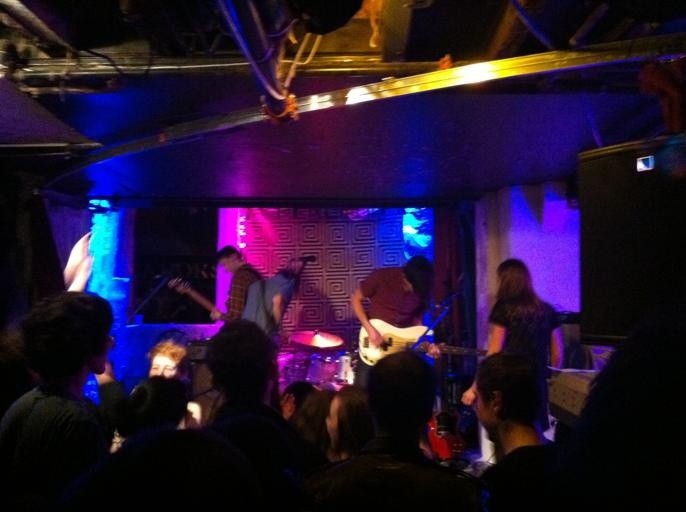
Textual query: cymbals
290,331,343,348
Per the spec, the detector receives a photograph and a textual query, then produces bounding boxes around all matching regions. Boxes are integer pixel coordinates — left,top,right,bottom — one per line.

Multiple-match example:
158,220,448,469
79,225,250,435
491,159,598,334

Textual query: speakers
575,133,686,346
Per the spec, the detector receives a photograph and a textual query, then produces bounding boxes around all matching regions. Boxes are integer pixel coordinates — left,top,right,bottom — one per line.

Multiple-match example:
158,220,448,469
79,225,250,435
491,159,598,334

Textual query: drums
306,351,341,384
276,351,310,386
333,350,368,389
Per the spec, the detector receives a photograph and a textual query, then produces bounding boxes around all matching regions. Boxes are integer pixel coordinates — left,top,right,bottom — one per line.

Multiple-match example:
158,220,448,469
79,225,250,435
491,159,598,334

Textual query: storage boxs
548,371,589,415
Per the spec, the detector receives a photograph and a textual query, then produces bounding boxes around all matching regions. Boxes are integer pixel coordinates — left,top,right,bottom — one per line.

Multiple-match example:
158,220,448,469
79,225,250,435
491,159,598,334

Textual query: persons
209,245,264,324
2,290,686,511
461,259,565,463
351,257,446,389
243,271,300,335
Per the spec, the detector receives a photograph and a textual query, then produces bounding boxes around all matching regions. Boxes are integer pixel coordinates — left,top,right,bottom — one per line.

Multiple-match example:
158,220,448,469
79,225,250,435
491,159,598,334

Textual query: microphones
293,253,318,264
156,271,183,279
437,290,459,309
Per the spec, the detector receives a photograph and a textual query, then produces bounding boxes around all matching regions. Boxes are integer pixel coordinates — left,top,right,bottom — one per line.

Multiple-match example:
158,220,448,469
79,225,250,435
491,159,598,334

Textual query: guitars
358,318,488,366
168,278,220,314
